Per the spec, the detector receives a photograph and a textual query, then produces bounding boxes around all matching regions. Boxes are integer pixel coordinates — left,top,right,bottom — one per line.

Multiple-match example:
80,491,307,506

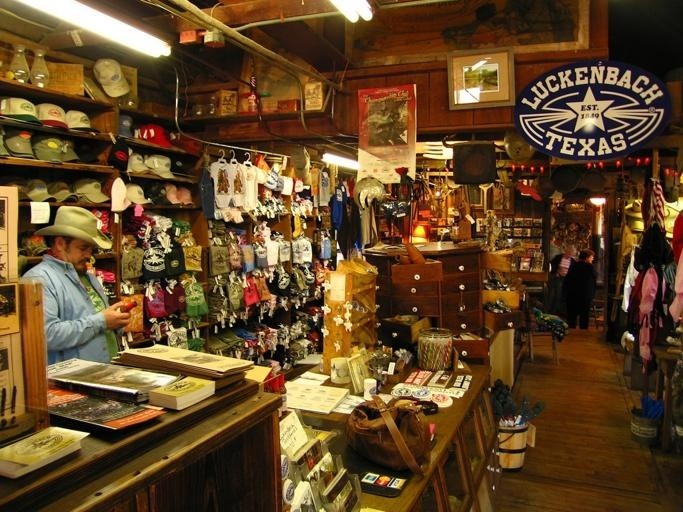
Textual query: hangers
215,148,256,172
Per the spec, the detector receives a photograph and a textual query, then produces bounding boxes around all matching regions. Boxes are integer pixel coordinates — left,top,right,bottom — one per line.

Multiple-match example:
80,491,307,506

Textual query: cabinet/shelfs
361,246,524,365
0,41,209,351
473,217,549,291
0,377,282,512
650,345,683,451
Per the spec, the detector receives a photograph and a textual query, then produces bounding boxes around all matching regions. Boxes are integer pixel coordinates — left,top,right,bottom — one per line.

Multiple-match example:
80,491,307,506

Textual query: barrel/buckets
498,423,529,473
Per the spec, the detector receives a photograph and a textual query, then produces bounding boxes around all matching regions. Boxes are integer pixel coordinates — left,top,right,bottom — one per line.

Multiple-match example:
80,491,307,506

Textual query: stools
528,328,559,366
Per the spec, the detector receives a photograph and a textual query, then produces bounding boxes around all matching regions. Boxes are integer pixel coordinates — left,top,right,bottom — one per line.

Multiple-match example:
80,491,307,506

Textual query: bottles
416,328,455,372
27,47,49,90
8,42,30,84
247,75,263,113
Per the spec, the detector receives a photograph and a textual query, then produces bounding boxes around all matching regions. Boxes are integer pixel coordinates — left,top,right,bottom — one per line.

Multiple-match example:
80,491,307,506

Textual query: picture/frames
447,46,515,111
470,183,515,216
344,1,609,78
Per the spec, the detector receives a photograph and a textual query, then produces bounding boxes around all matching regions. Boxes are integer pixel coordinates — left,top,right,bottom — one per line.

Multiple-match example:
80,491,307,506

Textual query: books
0,425,82,479
279,381,350,416
42,342,254,432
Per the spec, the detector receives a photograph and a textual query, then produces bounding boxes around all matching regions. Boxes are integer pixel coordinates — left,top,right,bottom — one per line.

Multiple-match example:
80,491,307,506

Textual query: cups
329,357,351,384
206,95,218,118
191,104,206,118
363,378,378,402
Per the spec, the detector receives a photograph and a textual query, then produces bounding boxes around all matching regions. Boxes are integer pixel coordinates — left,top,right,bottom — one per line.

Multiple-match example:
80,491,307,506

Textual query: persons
563,249,599,332
17,233,133,369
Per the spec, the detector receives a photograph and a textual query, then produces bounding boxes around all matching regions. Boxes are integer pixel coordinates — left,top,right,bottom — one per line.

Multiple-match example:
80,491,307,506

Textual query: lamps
326,0,373,24
317,149,357,172
13,0,176,59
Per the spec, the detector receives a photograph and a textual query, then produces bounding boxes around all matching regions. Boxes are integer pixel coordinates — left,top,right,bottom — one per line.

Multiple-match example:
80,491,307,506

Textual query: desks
283,365,501,512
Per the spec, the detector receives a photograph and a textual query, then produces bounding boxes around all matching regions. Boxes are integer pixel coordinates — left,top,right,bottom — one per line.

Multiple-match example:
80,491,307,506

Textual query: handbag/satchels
344,398,428,466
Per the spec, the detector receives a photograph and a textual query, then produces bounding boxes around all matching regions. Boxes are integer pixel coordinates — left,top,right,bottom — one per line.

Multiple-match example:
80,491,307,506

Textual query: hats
0,96,195,207
18,231,50,255
92,57,131,99
35,205,113,252
